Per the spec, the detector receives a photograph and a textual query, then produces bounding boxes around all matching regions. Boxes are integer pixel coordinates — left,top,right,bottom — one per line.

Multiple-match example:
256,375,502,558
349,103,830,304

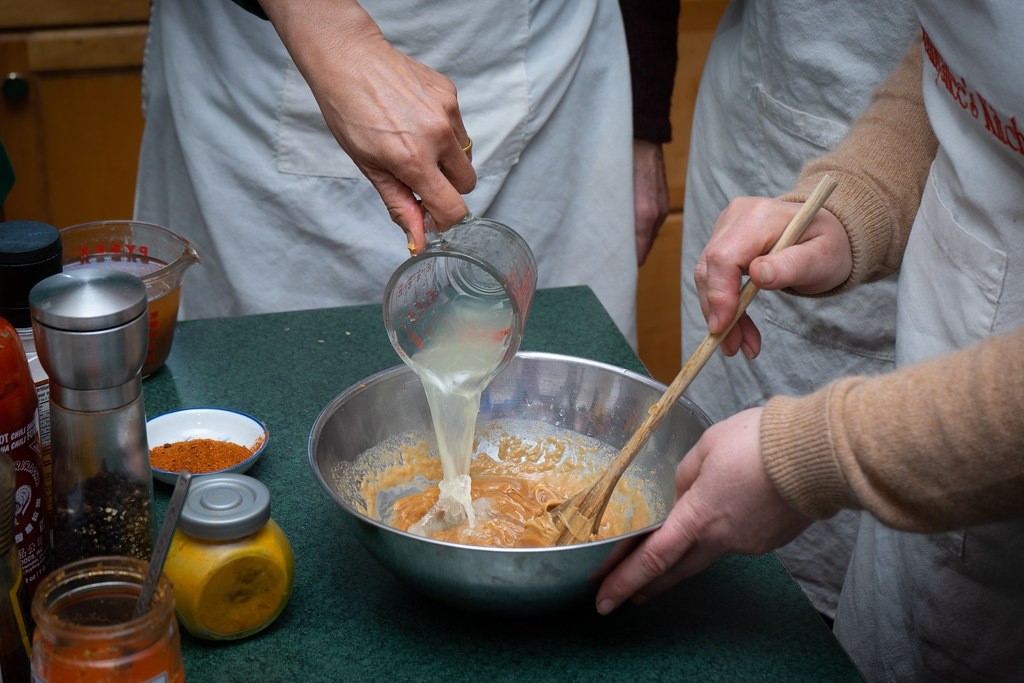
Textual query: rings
458,136,474,152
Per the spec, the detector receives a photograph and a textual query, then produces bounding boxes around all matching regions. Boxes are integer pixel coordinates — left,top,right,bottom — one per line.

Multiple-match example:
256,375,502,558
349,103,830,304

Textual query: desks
2,279,867,683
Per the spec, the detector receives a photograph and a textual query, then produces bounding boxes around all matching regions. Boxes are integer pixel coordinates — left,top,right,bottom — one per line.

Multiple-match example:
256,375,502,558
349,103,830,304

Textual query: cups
379,204,536,405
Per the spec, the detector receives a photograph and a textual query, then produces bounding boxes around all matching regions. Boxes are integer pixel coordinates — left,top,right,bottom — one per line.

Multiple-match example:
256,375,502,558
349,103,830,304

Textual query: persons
131,1,684,370
231,1,478,258
597,0,1024,682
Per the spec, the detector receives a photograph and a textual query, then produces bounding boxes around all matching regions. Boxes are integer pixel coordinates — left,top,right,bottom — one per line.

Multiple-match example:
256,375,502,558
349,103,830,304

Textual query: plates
147,409,276,489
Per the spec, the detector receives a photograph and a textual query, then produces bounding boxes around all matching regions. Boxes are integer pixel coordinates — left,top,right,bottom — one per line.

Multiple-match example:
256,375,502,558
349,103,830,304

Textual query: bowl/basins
307,347,719,615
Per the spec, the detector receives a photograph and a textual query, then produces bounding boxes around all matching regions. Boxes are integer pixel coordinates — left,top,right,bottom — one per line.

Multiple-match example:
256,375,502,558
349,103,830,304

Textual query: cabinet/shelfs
0,0,150,253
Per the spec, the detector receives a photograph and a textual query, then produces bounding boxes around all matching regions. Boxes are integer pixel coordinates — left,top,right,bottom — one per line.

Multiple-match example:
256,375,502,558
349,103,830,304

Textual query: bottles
27,555,186,682
164,472,295,645
1,218,66,681
34,268,167,564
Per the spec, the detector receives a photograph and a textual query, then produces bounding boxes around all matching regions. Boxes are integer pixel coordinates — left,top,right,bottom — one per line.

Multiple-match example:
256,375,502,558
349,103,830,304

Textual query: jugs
55,217,198,383
